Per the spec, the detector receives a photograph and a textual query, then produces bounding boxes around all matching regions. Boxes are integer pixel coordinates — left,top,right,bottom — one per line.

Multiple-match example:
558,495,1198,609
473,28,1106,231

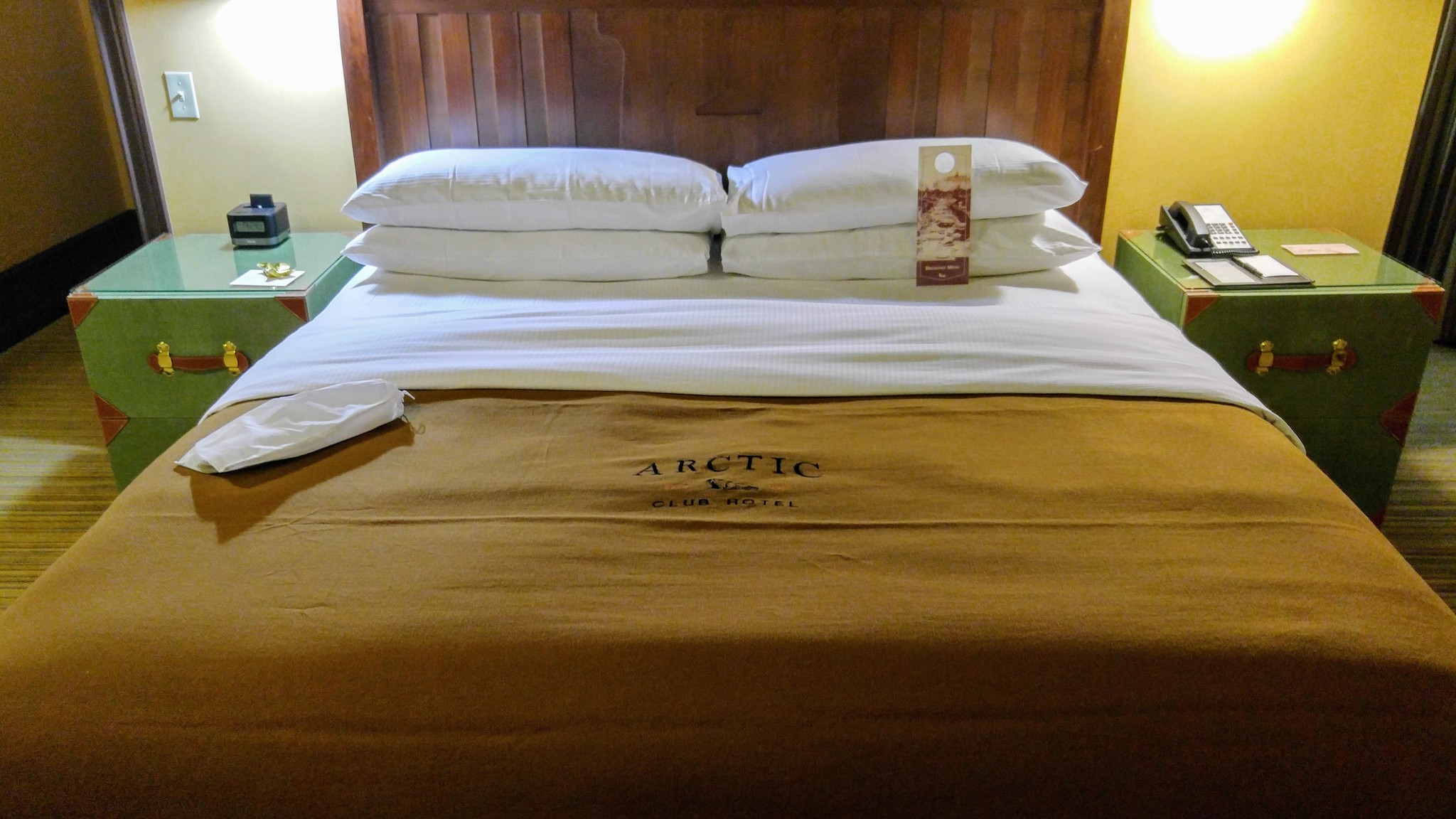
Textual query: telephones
1159,204,1255,256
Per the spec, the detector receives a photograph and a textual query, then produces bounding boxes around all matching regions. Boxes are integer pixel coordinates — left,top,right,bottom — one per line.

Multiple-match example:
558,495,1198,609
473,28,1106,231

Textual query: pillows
338,226,716,283
340,144,726,236
721,138,1088,239
721,209,1104,281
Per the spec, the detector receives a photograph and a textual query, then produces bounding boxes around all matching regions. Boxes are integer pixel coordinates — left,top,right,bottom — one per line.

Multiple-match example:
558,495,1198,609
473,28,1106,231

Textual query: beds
0,1,1456,819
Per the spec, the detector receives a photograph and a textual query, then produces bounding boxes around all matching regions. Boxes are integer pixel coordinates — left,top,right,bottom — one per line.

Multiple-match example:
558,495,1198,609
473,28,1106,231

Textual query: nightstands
1114,227,1447,530
66,233,364,494
